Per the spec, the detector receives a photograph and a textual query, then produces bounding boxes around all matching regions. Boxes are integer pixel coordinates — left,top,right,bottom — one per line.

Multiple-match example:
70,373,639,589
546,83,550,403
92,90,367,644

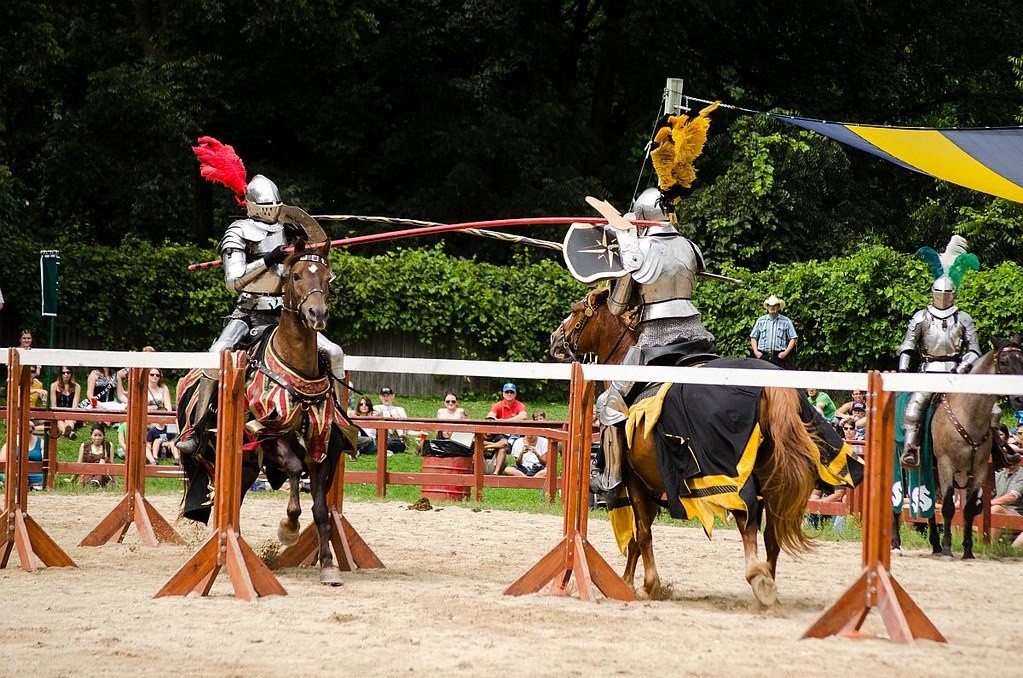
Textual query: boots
358,436,370,447
176,378,220,453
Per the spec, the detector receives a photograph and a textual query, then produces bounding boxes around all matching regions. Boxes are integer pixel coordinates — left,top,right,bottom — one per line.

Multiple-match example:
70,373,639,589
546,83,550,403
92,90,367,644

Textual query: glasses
359,403,370,406
31,370,35,373
505,390,514,394
1017,431,1022,435
62,372,70,374
150,374,160,377
446,400,456,403
844,427,854,430
1013,443,1017,446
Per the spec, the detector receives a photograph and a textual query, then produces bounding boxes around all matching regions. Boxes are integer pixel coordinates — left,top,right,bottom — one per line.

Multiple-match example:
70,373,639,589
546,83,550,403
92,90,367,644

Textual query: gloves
264,245,290,269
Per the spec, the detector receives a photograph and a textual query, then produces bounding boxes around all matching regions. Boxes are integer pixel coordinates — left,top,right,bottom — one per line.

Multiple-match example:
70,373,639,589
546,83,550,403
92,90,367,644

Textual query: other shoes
992,452,1021,471
589,474,622,504
154,458,159,464
902,454,915,469
174,459,179,464
64,425,77,441
91,480,100,490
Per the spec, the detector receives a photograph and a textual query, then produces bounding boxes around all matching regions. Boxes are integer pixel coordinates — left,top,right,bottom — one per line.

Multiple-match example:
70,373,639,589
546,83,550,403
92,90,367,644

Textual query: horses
172,233,362,588
547,282,828,622
889,326,1023,563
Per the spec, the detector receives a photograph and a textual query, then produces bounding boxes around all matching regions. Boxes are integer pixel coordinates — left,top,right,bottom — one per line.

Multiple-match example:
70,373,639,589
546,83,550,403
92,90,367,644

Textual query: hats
485,416,496,420
503,383,516,392
764,295,785,309
381,388,393,394
852,401,866,412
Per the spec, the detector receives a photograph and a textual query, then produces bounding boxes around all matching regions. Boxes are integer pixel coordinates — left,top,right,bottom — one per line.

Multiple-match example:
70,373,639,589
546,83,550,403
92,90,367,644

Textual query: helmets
632,101,719,236
915,235,980,310
192,136,283,223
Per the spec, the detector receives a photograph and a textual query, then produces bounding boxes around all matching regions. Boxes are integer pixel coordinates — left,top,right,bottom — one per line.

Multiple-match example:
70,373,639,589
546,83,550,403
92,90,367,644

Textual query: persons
0,288,180,492
895,235,983,470
588,100,722,504
749,295,799,368
800,388,1023,549
345,382,562,478
175,135,371,454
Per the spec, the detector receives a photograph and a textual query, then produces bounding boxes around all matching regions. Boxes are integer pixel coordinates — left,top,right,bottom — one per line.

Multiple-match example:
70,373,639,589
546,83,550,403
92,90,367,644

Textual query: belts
761,350,785,356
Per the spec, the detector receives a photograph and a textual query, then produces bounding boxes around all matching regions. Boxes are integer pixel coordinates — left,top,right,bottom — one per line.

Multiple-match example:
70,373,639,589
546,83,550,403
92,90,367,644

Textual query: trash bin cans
420,439,472,501
